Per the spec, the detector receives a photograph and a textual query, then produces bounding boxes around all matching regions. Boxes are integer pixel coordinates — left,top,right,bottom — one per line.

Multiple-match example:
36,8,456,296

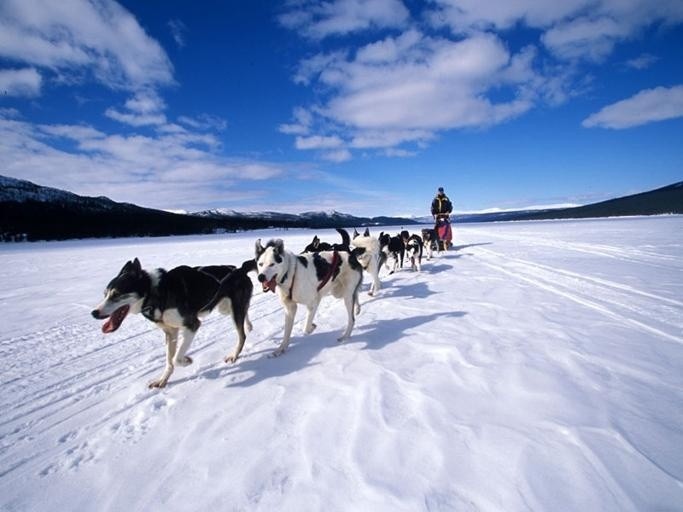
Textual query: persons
430,187,453,251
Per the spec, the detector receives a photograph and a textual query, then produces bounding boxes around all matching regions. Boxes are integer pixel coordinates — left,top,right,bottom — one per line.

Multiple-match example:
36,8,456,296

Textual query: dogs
90,257,258,389
254,228,424,357
435,214,450,254
421,229,440,261
